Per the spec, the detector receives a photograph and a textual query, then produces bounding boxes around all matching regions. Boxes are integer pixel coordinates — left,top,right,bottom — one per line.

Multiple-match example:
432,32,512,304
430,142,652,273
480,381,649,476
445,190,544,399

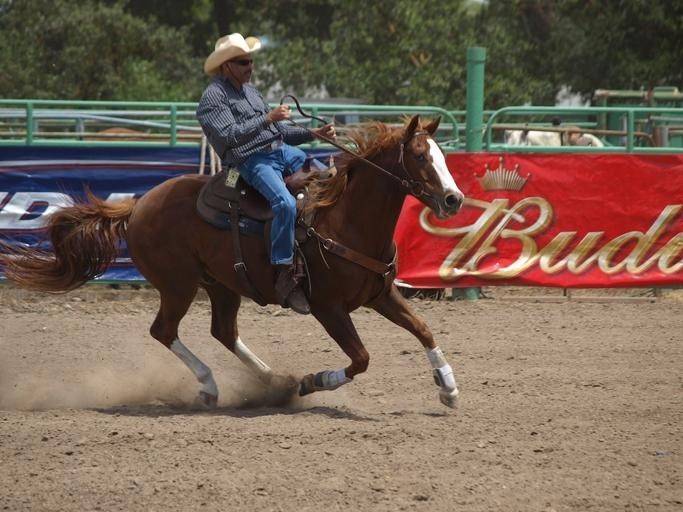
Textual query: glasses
226,60,252,66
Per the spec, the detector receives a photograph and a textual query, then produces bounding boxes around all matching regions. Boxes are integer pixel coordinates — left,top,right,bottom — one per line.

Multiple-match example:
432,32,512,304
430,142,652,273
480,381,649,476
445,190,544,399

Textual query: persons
196,33,338,314
547,117,565,146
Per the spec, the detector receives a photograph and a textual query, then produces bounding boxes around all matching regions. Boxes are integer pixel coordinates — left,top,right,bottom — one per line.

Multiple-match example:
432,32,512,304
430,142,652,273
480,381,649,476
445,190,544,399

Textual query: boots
274,265,310,314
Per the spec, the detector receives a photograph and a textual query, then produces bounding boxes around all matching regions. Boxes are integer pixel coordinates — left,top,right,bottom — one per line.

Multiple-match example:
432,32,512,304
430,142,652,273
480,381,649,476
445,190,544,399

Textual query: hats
203,33,261,74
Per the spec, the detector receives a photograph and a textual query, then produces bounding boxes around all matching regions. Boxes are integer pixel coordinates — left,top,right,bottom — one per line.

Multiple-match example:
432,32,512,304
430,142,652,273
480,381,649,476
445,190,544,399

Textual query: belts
263,140,284,153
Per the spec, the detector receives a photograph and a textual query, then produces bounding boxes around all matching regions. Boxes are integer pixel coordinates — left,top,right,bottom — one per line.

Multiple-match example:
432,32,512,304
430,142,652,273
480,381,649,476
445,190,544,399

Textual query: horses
0,112,467,413
